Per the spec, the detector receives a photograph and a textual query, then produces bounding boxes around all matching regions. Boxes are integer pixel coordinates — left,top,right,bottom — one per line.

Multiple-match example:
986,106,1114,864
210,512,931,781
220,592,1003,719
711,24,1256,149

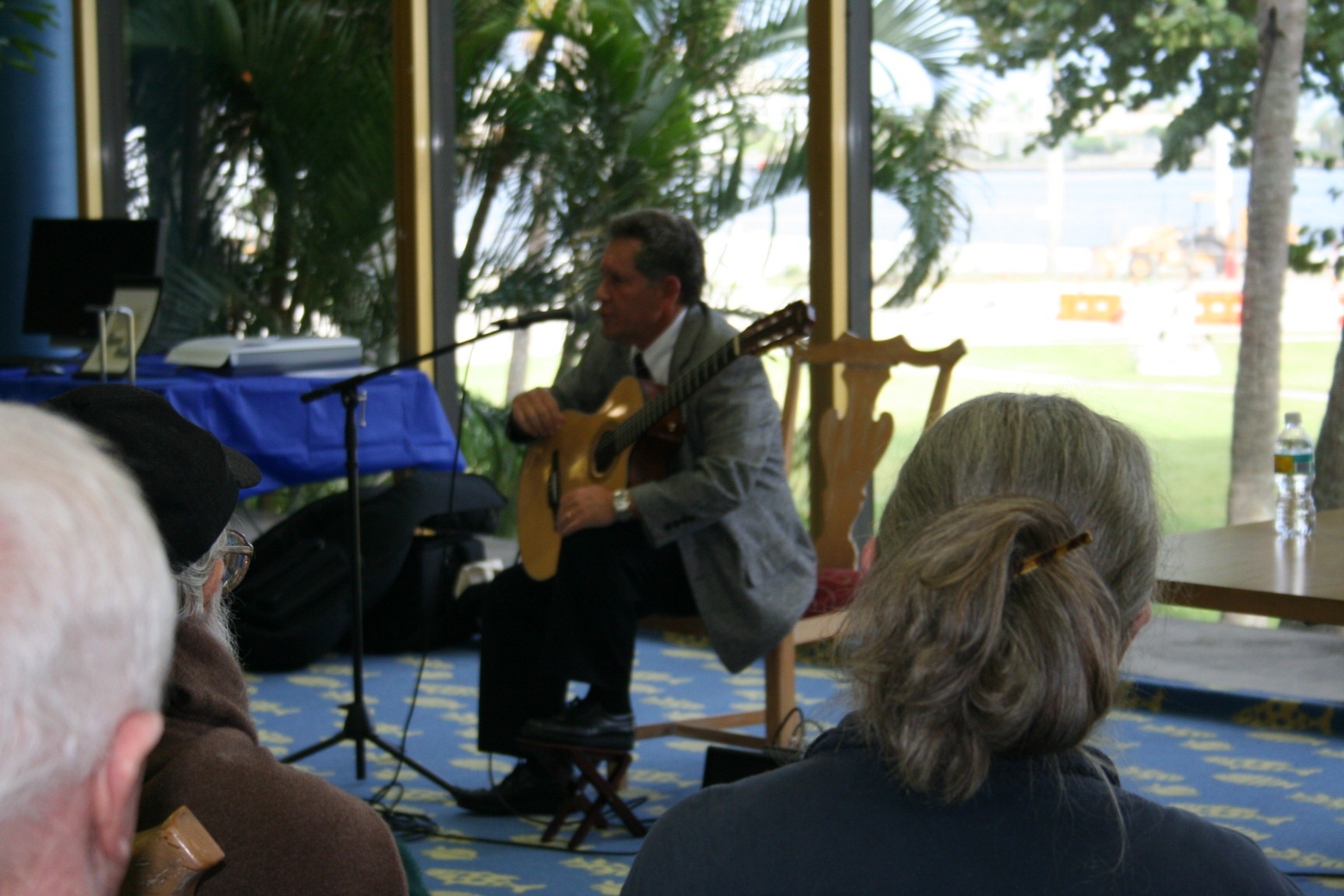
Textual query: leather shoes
457,764,575,814
526,696,634,749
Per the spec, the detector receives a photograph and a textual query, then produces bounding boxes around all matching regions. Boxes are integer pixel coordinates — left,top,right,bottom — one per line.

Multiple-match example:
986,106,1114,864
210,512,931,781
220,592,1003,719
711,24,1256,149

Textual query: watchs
612,490,634,525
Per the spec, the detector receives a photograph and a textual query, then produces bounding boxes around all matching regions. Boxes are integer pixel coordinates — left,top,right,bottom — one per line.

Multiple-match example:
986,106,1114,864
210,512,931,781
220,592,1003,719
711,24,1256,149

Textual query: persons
0,384,429,896
455,209,818,817
619,391,1302,896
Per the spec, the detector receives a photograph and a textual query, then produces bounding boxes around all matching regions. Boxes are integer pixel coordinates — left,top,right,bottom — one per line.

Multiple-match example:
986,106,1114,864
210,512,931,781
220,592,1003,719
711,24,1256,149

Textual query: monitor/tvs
21,217,157,337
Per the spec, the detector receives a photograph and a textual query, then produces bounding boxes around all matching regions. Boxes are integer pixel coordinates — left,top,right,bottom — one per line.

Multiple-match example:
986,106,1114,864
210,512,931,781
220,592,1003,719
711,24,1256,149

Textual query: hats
33,383,262,573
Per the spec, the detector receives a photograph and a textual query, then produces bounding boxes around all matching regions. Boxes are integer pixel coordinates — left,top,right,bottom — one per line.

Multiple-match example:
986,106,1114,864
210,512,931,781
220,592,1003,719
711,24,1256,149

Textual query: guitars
516,298,818,582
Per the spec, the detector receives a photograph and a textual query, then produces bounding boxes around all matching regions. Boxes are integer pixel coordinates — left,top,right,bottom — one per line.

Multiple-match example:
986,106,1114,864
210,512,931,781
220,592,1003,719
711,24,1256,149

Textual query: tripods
275,328,526,817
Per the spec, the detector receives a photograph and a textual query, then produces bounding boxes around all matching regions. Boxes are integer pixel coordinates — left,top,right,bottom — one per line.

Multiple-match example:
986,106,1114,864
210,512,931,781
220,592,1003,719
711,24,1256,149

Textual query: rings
564,511,574,524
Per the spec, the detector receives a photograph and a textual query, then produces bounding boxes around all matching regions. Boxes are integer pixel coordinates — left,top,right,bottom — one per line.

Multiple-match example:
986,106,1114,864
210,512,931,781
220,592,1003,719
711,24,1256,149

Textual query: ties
634,353,651,388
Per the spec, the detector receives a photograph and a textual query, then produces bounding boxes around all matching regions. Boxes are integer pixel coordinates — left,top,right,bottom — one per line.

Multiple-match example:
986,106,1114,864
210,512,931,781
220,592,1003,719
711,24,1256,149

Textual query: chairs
627,334,968,771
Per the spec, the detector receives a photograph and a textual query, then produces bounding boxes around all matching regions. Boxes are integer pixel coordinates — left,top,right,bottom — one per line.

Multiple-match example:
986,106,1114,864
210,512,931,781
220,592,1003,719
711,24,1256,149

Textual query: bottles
1272,414,1318,536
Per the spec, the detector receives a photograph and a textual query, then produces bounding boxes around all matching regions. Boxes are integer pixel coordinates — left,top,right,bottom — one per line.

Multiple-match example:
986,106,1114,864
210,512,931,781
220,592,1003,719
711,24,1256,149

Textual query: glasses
219,528,254,597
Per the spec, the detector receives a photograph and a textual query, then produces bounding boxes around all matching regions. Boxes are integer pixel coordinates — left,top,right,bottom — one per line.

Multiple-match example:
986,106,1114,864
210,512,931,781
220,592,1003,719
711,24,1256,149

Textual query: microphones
495,301,597,326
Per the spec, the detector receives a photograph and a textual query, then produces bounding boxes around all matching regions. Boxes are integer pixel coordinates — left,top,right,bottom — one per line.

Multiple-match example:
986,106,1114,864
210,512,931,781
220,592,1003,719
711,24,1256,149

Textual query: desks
1158,508,1344,632
0,349,465,498
